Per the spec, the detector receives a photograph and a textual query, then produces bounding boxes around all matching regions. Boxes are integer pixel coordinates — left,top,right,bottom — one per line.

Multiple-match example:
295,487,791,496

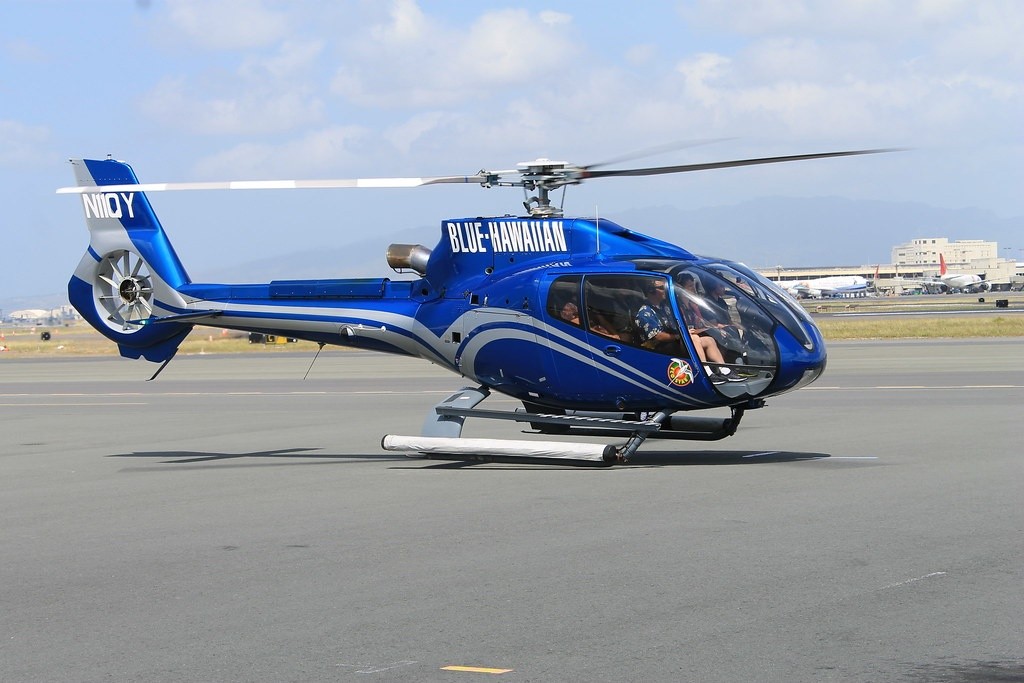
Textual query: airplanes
767,252,1017,301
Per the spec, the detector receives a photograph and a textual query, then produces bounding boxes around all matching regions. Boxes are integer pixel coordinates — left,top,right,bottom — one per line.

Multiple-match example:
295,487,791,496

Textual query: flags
874,267,879,280
940,253,948,275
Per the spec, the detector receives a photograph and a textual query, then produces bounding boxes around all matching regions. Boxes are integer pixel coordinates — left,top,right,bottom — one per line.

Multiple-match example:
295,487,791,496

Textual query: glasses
655,285,665,290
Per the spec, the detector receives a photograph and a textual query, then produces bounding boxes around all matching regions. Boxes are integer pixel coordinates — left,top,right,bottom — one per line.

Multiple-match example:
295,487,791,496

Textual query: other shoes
734,364,759,376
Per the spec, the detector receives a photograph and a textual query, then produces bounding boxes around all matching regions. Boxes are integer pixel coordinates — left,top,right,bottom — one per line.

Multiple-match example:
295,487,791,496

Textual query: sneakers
718,369,748,381
708,372,728,384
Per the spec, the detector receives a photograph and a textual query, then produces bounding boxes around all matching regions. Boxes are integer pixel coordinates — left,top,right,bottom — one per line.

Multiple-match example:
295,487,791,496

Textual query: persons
560,274,759,384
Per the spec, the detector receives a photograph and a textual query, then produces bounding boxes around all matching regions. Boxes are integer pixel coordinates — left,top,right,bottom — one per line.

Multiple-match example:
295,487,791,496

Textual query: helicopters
54,147,911,467
